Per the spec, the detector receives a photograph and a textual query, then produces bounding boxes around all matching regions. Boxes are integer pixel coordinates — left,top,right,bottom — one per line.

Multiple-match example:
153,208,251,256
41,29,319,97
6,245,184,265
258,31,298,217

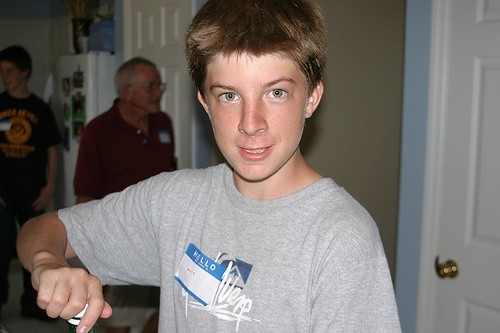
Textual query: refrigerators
44,51,116,213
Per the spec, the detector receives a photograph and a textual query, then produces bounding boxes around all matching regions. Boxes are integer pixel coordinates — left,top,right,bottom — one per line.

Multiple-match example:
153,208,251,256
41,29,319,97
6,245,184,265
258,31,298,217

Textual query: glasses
136,82,166,92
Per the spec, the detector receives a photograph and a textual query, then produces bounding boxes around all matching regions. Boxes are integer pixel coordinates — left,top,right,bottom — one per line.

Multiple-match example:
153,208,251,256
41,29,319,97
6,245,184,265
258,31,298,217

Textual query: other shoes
20,293,58,322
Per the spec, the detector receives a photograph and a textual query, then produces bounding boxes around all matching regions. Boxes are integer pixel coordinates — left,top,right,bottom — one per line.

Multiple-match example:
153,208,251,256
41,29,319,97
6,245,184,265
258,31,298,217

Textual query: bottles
66,304,95,333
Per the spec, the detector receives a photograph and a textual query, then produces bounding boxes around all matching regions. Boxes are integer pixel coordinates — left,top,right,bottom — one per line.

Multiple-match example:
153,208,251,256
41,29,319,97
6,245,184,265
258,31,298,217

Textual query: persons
1,44,63,323
74,57,178,333
16,0,401,333
